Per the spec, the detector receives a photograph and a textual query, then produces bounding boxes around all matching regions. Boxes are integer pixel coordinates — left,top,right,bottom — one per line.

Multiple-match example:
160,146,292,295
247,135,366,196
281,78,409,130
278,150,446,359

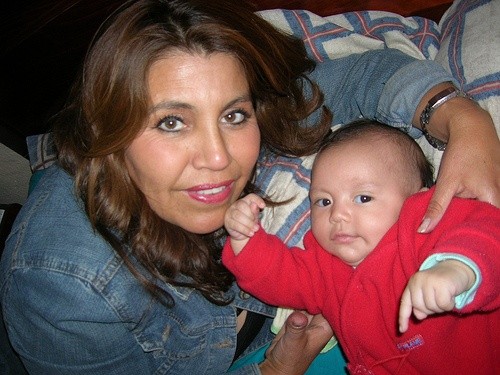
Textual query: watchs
419,85,479,150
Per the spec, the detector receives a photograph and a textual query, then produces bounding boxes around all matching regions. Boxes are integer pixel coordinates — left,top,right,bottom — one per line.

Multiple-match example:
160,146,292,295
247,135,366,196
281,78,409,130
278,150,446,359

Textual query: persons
0,0,500,375
220,119,500,375
